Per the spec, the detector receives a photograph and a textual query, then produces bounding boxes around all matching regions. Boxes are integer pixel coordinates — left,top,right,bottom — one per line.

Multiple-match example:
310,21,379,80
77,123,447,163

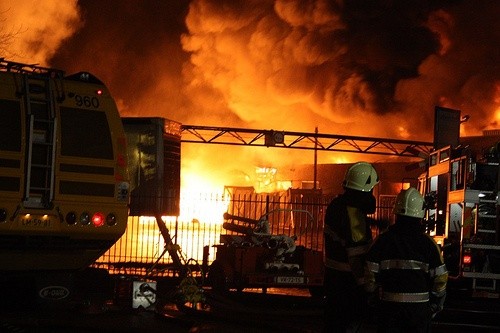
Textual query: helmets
395,187,425,218
345,162,378,192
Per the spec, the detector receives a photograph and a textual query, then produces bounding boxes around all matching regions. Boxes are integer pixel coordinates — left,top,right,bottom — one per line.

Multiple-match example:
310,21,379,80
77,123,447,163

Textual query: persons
322,162,376,333
369,187,448,333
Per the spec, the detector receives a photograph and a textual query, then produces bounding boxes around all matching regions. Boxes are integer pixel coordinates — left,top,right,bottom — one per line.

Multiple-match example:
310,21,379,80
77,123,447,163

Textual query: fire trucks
417,141,499,301
0,59,130,309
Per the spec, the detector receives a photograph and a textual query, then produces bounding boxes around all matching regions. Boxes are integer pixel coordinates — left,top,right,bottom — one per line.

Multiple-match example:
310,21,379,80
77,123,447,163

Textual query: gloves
430,302,443,319
